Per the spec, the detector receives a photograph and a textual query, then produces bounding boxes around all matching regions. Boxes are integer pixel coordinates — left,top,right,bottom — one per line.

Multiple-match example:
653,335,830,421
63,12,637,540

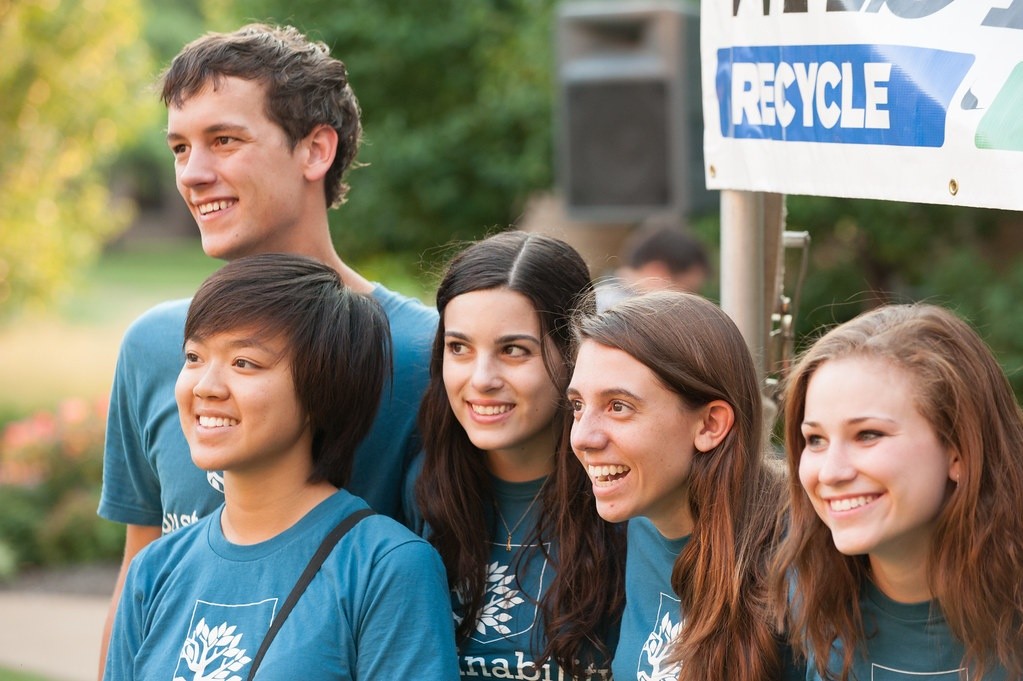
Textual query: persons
101,253,461,681
565,285,792,681
97,21,441,681
618,228,710,292
401,228,626,681
759,300,1023,681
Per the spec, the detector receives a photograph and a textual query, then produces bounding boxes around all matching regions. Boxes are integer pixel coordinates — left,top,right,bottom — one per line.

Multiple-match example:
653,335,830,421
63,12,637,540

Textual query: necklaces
491,473,549,552
